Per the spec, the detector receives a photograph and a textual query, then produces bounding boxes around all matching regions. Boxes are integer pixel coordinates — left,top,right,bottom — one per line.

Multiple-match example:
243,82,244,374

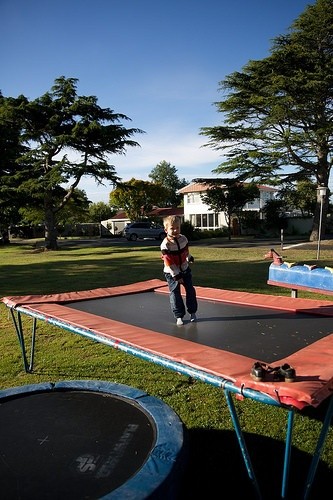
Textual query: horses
264,249,284,266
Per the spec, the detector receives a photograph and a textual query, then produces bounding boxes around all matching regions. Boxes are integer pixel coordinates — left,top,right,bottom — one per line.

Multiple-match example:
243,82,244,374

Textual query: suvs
124,222,166,241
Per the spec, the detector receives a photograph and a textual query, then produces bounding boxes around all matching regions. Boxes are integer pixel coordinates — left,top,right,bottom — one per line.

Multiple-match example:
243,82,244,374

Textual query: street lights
317,182,328,260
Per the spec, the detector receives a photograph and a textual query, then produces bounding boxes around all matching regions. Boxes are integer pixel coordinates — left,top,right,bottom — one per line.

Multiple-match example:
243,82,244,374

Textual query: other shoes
250,362,267,381
273,364,296,382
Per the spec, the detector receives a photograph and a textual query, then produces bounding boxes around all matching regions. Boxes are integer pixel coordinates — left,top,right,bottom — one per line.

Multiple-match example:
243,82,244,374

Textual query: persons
160,215,197,325
